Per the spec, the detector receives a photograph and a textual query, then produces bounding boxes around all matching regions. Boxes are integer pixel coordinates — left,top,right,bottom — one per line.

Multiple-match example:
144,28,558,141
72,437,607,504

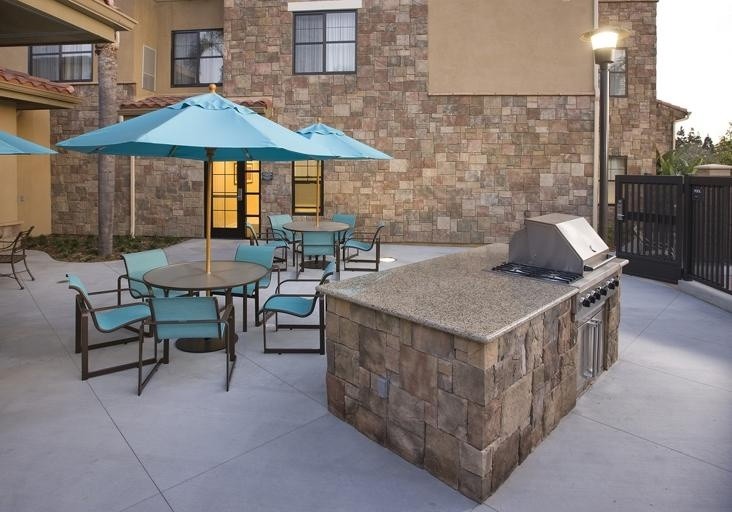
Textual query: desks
282,218,350,270
143,259,269,354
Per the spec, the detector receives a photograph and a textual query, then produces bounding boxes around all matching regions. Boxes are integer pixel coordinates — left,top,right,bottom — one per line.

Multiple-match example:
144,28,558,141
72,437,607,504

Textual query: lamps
583,26,619,241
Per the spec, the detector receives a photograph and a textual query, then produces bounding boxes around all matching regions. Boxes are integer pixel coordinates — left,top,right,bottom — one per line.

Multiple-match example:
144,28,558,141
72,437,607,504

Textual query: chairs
329,213,359,261
292,231,341,282
244,220,290,273
210,243,279,327
339,221,385,273
67,271,158,382
257,261,336,356
136,295,237,397
117,248,200,338
265,212,302,266
0,224,35,291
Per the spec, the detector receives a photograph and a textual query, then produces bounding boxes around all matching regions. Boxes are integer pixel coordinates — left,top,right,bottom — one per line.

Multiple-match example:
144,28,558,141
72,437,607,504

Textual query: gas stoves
492,263,583,286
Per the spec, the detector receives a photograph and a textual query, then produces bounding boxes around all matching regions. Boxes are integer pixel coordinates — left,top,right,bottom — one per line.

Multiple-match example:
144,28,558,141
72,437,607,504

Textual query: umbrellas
55,84,340,353
0,130,58,155
292,118,394,262
